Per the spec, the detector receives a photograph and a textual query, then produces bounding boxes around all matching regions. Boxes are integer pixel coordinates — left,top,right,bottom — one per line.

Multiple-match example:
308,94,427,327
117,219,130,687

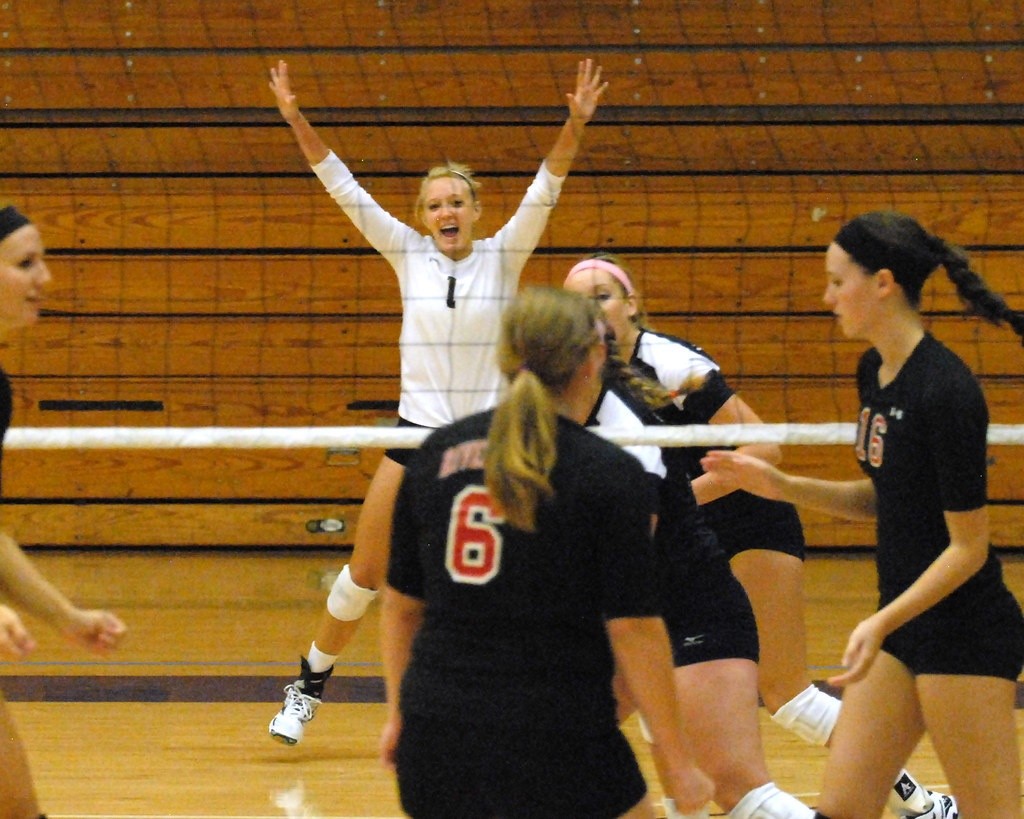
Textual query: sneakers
902,791,959,819
269,686,321,746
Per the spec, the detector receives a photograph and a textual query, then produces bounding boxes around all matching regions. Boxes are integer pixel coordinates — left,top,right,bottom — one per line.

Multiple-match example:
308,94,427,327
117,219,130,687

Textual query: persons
699,208,1024,818
379,283,717,819
268,54,611,748
563,249,960,819
0,203,130,818
